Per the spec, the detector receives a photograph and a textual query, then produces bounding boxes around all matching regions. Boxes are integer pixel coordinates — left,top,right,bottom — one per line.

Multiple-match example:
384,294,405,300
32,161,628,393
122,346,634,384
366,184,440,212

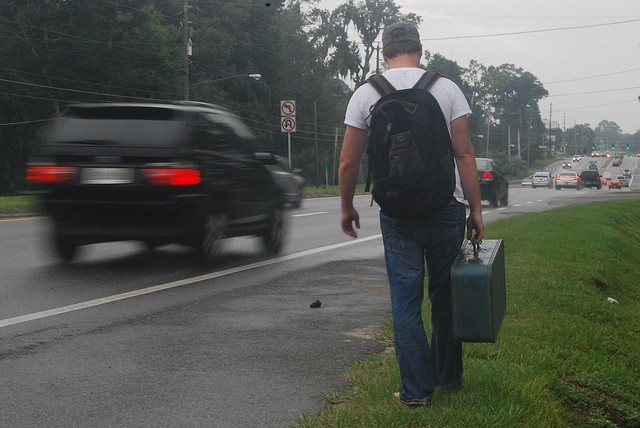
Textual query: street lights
181,3,262,99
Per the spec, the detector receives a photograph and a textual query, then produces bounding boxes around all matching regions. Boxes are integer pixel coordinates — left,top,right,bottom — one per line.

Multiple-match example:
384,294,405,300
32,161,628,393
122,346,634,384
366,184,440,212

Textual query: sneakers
394,392,431,407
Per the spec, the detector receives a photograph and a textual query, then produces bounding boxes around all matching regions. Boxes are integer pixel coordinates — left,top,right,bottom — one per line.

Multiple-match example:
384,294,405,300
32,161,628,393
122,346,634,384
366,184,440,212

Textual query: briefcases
450,237,507,343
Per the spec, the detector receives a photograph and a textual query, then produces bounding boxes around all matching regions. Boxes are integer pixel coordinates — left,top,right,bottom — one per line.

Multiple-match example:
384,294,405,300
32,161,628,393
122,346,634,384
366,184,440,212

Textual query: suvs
26,100,292,264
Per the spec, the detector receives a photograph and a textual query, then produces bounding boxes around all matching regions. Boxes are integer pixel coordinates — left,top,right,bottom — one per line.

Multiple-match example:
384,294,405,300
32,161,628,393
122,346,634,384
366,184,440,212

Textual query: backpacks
361,69,456,215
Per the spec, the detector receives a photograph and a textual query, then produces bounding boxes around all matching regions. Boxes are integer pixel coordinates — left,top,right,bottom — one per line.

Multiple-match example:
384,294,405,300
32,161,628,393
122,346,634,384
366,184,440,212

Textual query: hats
381,21,420,48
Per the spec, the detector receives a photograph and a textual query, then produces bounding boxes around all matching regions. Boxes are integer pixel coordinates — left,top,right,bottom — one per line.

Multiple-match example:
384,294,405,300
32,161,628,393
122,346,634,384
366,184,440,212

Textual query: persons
338,22,485,406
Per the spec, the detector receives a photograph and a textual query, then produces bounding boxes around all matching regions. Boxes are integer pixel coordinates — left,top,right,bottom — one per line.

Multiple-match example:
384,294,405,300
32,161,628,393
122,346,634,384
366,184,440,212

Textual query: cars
624,169,631,176
589,161,598,169
581,170,602,190
613,152,623,165
532,172,554,189
258,153,304,209
603,172,611,179
591,151,599,157
620,179,629,187
476,158,508,207
609,179,621,189
600,177,608,185
563,162,572,168
616,175,626,179
573,155,580,162
522,178,532,188
556,172,583,190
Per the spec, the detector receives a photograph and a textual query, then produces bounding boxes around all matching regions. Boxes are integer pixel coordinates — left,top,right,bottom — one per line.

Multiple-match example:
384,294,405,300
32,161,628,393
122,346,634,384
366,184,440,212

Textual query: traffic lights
592,145,595,151
626,144,629,151
612,144,614,151
